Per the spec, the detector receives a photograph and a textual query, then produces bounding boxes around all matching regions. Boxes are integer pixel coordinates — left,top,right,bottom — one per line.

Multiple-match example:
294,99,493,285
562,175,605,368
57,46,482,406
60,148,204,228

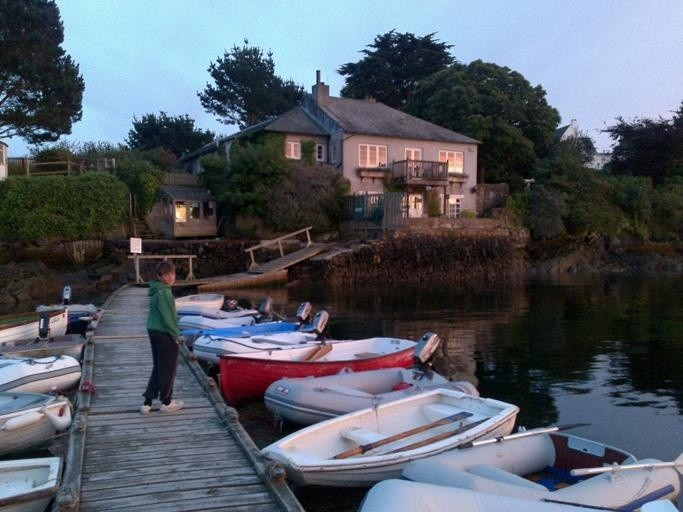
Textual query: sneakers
140,405,151,415
160,399,184,413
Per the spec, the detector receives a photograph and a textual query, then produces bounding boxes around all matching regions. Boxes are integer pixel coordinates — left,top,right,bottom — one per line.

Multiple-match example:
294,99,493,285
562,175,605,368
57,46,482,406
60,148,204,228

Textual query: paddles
332,411,473,459
377,418,489,455
457,423,593,450
570,452,683,476
308,342,332,361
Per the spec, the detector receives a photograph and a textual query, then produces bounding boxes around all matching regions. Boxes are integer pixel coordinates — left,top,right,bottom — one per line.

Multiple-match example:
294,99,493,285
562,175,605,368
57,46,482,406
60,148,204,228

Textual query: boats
0,285,99,512
360,420,683,511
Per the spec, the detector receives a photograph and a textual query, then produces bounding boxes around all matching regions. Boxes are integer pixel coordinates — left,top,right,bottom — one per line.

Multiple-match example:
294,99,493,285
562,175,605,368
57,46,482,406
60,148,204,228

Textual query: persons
140,259,184,415
